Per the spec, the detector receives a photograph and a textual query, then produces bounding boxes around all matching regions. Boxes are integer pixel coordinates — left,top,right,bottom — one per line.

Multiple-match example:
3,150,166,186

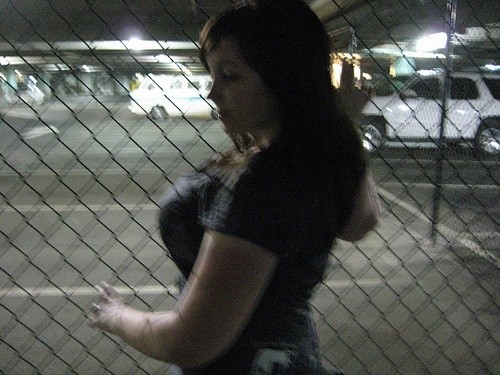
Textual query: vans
129,74,219,122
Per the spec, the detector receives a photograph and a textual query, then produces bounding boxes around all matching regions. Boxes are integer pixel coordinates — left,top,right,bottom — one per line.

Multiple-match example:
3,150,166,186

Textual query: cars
358,69,499,159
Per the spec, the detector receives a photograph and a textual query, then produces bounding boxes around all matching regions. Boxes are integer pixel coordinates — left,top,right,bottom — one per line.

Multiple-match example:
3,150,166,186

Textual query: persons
85,0,383,375
